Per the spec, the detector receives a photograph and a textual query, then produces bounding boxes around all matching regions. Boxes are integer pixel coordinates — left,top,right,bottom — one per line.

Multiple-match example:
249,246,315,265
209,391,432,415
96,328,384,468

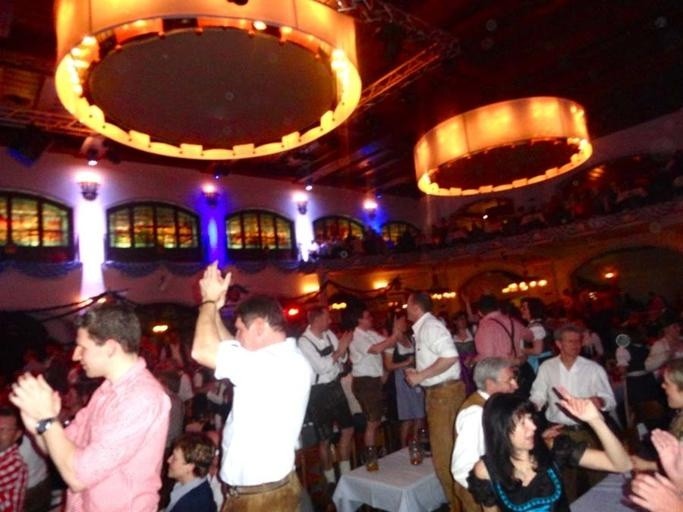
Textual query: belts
430,380,457,389
229,476,289,493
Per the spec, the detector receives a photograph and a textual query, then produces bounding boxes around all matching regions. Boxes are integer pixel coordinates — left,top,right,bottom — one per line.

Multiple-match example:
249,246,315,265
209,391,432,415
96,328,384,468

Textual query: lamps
48,0,364,163
410,88,594,203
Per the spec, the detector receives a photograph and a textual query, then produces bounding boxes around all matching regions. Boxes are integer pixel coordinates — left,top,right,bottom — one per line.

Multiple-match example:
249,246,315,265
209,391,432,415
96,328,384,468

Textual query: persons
307,147,682,260
1,257,683,511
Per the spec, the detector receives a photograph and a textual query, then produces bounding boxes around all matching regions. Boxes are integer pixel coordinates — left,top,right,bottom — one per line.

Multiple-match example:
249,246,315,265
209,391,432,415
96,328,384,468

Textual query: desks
338,444,451,512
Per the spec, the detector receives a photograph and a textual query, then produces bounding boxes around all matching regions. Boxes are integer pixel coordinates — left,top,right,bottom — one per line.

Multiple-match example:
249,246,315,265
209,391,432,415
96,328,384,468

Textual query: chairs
294,420,424,512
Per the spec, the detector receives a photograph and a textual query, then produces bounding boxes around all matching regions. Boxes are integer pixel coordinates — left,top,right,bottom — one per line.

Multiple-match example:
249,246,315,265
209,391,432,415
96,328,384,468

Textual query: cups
364,446,379,470
406,437,424,465
